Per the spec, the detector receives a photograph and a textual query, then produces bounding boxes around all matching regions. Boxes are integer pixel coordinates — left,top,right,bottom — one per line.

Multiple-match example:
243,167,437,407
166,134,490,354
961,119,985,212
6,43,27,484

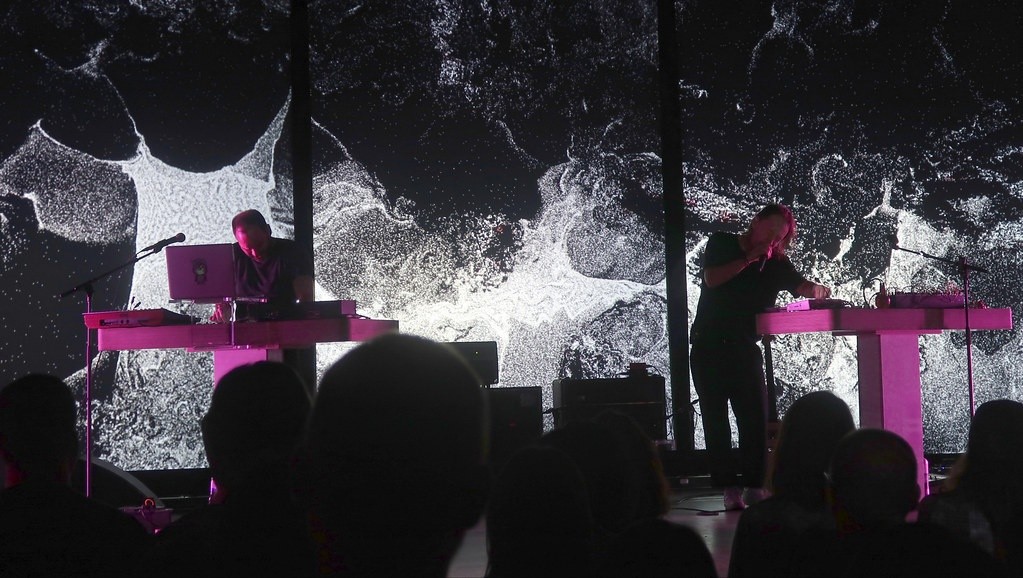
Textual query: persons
691,202,831,516
1,334,1023,577
208,210,315,323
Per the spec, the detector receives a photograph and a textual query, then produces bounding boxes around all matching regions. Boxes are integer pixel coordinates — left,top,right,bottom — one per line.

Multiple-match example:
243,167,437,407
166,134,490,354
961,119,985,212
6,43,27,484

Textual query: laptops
166,244,238,298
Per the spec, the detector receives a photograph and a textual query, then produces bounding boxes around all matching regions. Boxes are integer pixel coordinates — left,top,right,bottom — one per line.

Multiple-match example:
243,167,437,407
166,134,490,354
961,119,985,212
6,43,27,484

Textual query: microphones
143,233,185,251
758,246,771,271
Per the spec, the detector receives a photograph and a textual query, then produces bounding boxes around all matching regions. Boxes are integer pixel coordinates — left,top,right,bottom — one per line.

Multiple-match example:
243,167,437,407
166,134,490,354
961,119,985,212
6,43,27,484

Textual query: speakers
446,342,666,450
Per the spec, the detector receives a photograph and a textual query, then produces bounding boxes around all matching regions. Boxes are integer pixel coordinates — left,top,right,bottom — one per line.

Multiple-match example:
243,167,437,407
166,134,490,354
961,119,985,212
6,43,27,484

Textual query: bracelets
741,254,750,268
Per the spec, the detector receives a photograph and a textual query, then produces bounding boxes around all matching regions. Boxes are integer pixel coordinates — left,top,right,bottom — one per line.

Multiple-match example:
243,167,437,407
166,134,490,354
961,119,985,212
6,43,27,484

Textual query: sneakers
722,485,744,511
742,488,772,506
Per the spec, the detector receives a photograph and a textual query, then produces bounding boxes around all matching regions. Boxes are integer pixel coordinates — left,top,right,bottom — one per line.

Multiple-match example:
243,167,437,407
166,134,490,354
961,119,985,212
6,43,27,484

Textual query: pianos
754,281,1015,504
83,293,400,505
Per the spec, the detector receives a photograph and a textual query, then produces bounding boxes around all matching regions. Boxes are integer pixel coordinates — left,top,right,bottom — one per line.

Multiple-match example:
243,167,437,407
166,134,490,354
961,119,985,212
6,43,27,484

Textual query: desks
752,302,1014,507
93,312,394,516
